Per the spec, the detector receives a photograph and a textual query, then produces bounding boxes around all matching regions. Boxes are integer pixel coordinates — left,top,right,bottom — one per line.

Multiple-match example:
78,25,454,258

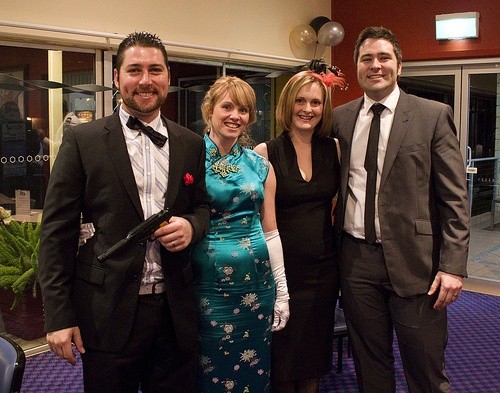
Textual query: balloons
318,21,345,47
309,16,331,43
292,24,316,47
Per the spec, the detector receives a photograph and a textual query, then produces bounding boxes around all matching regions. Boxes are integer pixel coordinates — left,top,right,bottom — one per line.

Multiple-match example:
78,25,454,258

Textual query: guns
97,207,172,264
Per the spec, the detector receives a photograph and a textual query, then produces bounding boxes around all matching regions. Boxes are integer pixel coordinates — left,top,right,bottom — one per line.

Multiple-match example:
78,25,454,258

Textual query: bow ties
125,115,167,147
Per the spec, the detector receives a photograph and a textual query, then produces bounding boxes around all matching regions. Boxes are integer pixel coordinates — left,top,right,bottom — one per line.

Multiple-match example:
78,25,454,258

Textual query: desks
12,210,43,224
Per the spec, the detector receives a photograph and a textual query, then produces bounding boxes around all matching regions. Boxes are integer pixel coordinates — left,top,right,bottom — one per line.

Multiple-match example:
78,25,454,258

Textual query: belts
139,283,166,295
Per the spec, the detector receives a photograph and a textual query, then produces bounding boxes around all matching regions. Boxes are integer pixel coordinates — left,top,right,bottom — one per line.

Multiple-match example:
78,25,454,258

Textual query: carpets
20,289,499,392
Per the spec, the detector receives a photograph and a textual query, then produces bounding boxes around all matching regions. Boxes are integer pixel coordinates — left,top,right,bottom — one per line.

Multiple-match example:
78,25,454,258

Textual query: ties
363,103,387,245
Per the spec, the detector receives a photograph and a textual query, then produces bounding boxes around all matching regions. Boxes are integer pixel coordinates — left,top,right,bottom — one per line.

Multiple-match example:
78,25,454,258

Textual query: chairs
334,303,349,373
0,335,25,393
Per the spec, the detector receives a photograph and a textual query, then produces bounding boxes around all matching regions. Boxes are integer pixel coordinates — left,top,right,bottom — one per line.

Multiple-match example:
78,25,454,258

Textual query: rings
171,241,176,247
453,294,458,297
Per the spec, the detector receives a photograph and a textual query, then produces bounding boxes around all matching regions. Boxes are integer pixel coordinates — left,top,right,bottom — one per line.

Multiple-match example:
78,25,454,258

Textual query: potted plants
0,213,46,341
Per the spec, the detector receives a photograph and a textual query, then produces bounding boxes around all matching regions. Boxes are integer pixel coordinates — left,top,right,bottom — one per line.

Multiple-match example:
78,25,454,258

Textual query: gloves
263,229,290,331
79,218,95,246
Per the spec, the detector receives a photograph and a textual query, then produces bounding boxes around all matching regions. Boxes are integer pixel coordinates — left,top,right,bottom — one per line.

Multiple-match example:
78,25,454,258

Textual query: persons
252,69,341,393
0,101,41,198
331,27,470,393
38,99,81,146
38,32,212,393
78,75,290,393
